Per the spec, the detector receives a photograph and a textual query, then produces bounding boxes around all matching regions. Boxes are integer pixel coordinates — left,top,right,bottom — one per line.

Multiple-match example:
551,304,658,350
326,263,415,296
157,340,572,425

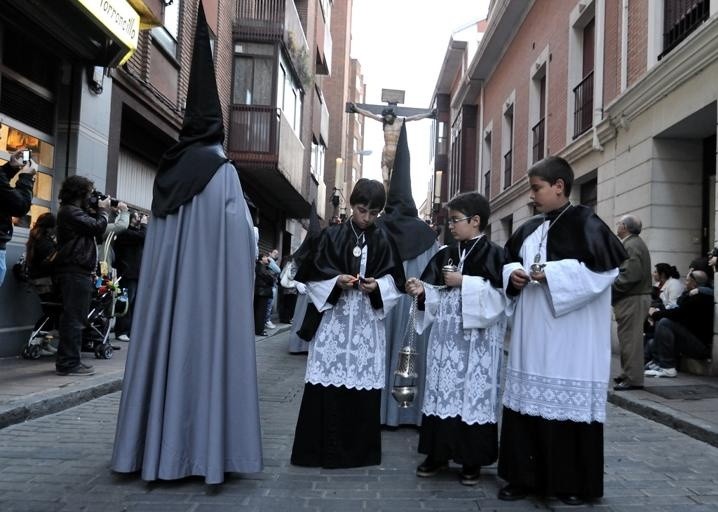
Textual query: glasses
447,215,472,222
688,266,698,282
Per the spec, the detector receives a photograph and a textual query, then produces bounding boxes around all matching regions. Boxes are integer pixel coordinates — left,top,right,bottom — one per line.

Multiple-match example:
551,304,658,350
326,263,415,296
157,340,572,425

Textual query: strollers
24,275,129,361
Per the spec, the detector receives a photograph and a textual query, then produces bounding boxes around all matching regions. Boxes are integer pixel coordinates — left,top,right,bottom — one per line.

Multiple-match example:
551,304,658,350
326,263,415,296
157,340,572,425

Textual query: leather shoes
498,480,549,501
416,454,450,476
460,463,482,485
614,376,623,382
559,488,585,505
54,364,95,376
612,380,643,390
79,361,95,370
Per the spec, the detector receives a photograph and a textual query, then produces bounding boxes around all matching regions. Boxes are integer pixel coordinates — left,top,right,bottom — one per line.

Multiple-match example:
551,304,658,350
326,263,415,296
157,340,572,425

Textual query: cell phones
22,150,31,166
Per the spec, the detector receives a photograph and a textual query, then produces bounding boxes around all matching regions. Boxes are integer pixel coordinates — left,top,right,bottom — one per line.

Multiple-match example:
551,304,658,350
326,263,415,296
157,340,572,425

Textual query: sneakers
266,322,276,328
117,334,130,342
644,365,678,378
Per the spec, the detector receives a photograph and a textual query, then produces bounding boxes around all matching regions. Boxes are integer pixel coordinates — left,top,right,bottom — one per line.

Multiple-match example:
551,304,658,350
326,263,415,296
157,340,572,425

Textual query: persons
1,148,150,376
641,249,718,377
611,215,654,392
348,102,438,192
110,119,265,486
256,155,631,504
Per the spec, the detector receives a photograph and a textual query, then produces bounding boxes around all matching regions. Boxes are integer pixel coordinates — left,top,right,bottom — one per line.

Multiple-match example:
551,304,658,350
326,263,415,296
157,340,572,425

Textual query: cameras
89,191,107,211
110,198,118,208
138,213,150,224
